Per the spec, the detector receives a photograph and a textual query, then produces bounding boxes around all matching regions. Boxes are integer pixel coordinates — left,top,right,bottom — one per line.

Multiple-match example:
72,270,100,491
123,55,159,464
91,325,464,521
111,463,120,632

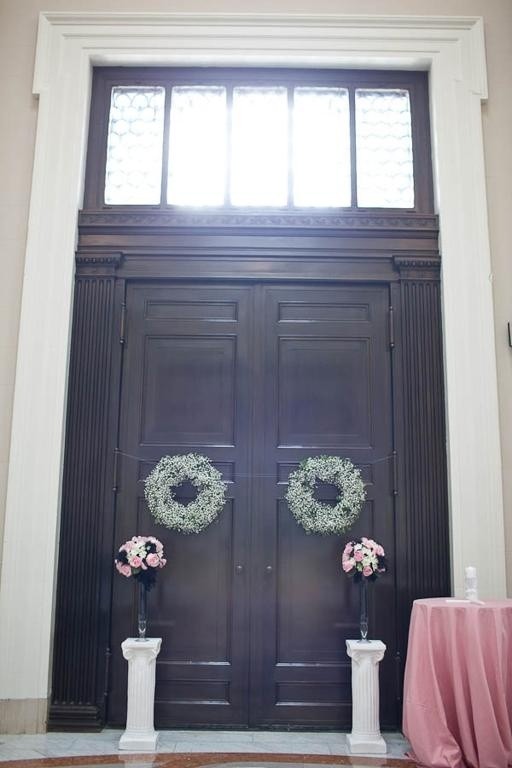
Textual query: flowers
284,454,368,536
114,534,168,592
341,538,389,582
142,450,227,536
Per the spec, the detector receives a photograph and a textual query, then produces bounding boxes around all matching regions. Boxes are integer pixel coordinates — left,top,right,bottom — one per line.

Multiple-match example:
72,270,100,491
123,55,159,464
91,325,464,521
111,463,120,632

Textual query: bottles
460,564,478,600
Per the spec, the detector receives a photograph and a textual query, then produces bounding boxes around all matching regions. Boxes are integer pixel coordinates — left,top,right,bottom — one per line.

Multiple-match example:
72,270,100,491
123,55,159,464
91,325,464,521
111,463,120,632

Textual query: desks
402,596,512,768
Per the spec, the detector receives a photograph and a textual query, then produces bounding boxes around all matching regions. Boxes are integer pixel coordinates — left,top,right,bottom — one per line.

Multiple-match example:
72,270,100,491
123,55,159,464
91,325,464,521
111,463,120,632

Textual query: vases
135,582,148,642
356,580,372,645
